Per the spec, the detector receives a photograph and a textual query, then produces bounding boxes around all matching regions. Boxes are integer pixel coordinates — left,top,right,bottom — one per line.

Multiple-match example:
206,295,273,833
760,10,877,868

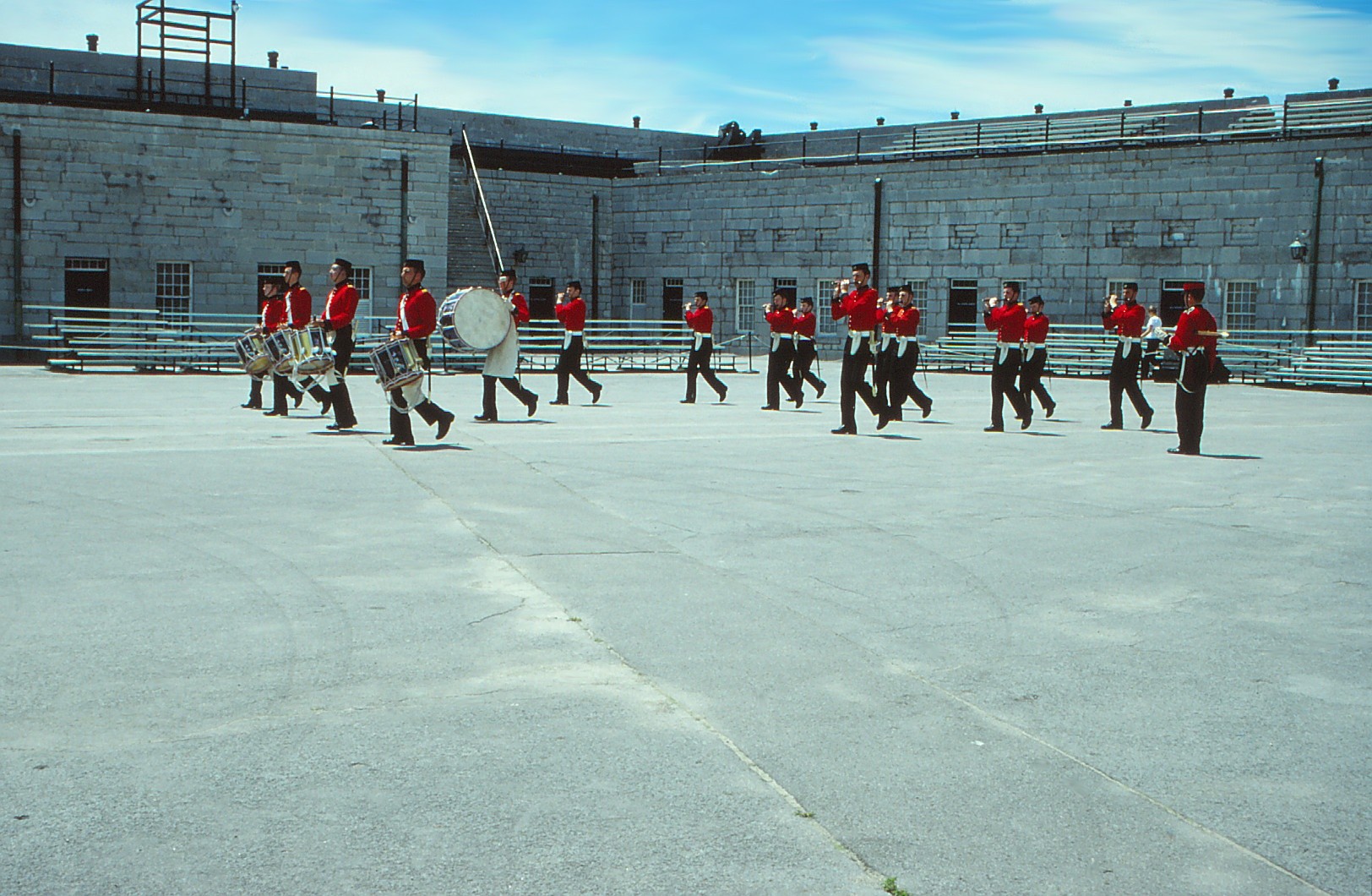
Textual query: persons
263,262,332,416
383,259,455,445
761,288,804,410
1138,305,1162,379
550,281,602,405
307,258,360,429
784,297,826,401
680,292,727,404
474,269,538,422
1014,294,1056,418
1154,282,1217,454
830,263,894,434
982,282,1034,432
240,277,304,408
1101,283,1154,429
873,285,933,421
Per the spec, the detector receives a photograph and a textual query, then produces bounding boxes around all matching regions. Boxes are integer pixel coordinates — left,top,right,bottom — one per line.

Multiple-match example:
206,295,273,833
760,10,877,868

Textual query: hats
1123,279,1137,289
1002,282,1019,288
771,262,915,298
1180,282,1204,296
1028,295,1042,305
263,258,581,289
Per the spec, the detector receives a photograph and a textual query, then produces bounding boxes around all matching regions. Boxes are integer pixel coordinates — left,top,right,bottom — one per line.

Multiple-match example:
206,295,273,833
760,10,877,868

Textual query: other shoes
719,386,728,402
679,397,695,403
762,379,1056,432
241,382,602,445
1102,421,1123,429
1141,408,1153,429
1167,446,1199,453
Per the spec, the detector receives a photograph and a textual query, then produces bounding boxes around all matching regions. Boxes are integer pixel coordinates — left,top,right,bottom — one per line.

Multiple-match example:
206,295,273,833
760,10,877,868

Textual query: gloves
990,297,997,307
1154,327,1168,342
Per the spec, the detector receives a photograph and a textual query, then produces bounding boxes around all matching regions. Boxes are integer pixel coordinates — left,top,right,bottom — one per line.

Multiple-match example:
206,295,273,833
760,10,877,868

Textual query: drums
234,333,272,374
290,326,336,375
438,286,511,354
261,328,295,372
368,338,424,391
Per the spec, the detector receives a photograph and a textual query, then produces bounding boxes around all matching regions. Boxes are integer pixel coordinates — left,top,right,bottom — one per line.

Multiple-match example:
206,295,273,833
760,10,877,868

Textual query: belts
1187,347,1204,351
771,332,792,339
1118,337,1141,343
896,336,916,341
694,332,711,338
794,334,813,341
998,342,1019,348
881,333,896,338
1023,343,1045,349
566,331,584,336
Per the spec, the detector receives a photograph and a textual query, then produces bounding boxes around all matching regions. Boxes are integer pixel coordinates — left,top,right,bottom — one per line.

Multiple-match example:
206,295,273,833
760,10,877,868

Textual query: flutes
831,282,861,285
1028,312,1038,314
682,303,696,309
1102,297,1130,301
797,309,803,312
892,301,903,305
981,298,1010,302
882,302,888,305
762,305,778,308
556,294,571,298
